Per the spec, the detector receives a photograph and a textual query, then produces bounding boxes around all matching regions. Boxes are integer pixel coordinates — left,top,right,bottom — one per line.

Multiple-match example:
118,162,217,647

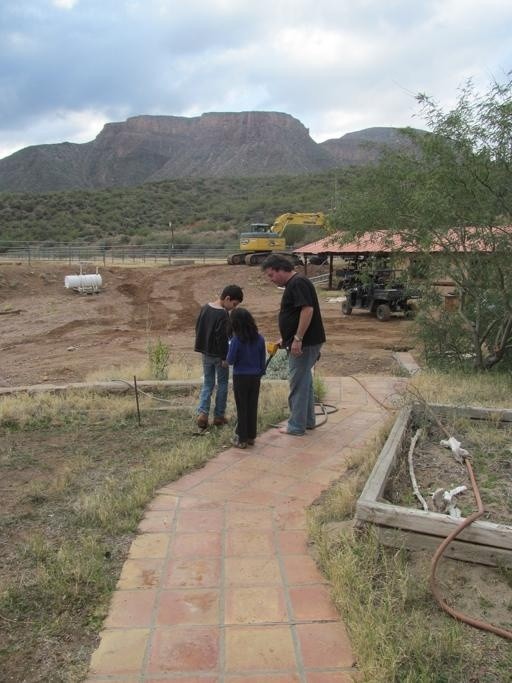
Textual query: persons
261,254,326,436
194,285,243,429
221,307,266,448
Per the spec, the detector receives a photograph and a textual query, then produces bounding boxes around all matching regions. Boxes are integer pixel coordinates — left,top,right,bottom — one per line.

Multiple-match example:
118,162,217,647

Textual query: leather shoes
277,424,316,437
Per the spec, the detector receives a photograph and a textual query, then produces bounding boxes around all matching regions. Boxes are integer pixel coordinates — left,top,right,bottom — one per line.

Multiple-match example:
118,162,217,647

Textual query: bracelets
293,334,304,341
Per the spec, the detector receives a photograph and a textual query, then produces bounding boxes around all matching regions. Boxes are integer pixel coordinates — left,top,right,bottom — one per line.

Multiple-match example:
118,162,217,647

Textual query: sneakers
230,435,256,450
213,416,229,426
196,412,209,427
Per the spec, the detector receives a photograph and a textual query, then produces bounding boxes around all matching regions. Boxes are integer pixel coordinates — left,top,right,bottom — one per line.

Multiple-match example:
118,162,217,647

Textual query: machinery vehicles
342,268,419,320
226,211,334,266
